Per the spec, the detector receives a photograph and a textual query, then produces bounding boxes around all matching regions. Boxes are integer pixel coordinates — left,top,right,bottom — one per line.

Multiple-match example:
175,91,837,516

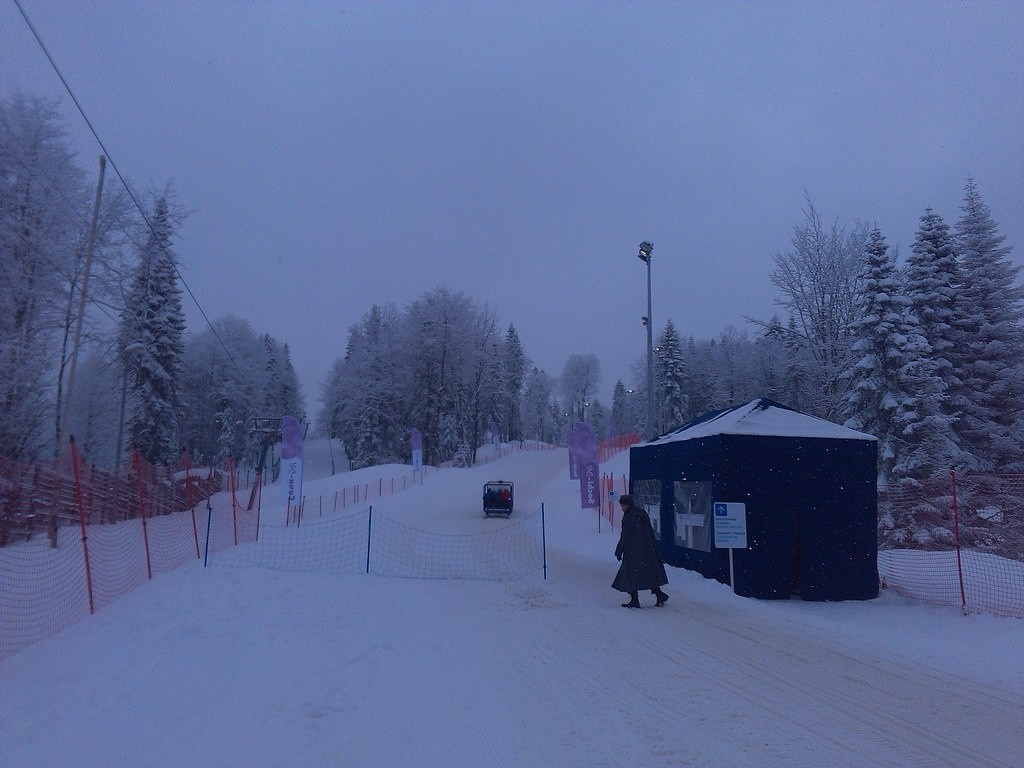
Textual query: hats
619,495,634,506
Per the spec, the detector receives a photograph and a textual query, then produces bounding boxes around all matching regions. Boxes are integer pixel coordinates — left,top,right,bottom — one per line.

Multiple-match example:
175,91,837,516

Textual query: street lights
639,240,655,442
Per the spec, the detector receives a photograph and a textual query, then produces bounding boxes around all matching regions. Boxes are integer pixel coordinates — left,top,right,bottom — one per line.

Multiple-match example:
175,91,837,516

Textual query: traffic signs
713,501,747,549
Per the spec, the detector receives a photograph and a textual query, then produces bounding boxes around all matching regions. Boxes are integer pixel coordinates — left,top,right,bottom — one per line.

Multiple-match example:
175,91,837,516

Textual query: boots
622,595,640,607
655,590,669,607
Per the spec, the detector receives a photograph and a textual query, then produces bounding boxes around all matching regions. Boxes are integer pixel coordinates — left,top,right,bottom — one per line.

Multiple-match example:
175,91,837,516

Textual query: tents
629,398,880,602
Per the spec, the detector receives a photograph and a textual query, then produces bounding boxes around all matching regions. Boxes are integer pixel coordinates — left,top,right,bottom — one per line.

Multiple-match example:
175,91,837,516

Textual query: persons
612,495,669,608
483,488,510,504
